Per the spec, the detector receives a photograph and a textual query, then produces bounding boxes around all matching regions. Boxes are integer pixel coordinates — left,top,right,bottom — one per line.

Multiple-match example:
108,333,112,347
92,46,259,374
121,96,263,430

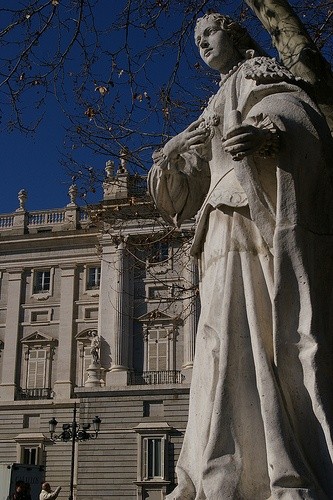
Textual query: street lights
42,400,102,500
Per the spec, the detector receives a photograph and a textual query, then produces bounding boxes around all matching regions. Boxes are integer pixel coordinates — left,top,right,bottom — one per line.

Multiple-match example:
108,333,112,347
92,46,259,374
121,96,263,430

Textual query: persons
7,481,27,500
148,13,333,500
91,332,99,364
39,483,61,500
